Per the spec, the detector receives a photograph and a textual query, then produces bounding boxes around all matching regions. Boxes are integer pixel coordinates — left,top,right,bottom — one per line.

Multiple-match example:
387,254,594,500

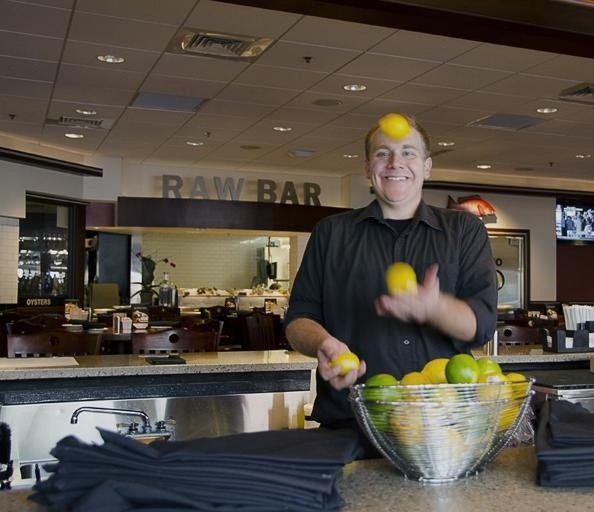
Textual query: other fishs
446,193,495,218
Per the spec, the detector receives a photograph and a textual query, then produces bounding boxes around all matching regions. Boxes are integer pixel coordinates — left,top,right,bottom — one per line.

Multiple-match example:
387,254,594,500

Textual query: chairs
495,300,571,347
0,304,225,357
239,306,281,352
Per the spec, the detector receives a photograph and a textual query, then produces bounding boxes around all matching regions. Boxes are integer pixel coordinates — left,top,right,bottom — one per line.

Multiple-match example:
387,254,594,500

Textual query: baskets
349,376,536,485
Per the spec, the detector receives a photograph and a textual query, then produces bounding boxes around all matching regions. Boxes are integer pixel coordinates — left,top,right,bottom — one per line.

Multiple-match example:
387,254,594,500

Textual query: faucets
71,406,152,433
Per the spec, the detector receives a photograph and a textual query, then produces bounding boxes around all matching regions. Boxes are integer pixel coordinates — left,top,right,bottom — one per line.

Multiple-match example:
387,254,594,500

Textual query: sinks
9,456,61,483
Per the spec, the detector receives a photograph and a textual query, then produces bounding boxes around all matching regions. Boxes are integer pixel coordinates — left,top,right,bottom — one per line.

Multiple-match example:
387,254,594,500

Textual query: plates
87,327,109,334
61,323,83,333
132,322,149,329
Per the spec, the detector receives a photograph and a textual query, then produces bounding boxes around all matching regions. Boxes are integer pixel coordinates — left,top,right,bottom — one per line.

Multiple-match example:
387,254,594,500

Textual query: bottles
158,271,171,307
20,271,64,297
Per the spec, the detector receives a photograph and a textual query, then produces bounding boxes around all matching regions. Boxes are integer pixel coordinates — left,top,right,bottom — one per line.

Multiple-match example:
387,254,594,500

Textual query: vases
139,289,151,305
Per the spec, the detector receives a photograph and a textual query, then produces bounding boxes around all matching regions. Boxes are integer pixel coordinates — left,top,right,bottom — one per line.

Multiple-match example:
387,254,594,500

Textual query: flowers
128,249,174,302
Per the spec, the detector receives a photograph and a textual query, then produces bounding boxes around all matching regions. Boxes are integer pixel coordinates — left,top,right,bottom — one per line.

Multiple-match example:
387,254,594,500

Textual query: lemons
378,113,408,139
328,351,361,379
384,260,417,297
362,352,533,482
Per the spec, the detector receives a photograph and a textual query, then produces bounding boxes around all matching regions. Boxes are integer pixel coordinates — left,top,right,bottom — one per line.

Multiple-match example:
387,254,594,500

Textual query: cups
122,317,132,334
112,315,120,335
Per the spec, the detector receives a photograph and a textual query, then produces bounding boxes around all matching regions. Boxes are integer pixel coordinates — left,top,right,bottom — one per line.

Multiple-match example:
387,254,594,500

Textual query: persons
282,112,500,431
562,207,594,237
